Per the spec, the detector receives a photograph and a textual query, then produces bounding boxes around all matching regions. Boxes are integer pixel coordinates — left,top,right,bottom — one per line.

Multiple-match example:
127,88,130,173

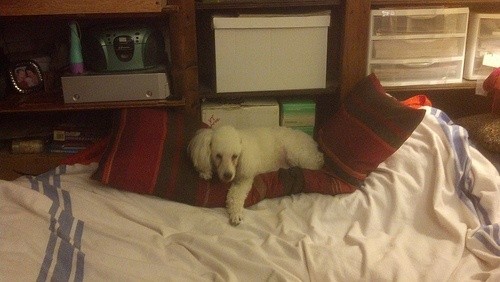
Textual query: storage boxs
211,11,332,94
369,6,469,86
200,98,280,128
278,99,316,137
464,10,500,80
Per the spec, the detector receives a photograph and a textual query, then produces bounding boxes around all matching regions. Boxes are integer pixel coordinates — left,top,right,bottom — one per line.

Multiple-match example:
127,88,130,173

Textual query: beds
0,109,500,282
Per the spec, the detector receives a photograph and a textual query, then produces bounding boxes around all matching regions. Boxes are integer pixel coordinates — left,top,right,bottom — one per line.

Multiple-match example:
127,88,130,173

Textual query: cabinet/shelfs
0,0,201,113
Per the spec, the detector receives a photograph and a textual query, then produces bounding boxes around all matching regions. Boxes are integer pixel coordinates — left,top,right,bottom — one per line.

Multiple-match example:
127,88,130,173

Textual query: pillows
455,113,500,155
315,73,426,188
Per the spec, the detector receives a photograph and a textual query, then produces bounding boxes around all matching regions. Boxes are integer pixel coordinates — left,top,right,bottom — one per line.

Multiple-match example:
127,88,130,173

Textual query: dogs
189,125,325,226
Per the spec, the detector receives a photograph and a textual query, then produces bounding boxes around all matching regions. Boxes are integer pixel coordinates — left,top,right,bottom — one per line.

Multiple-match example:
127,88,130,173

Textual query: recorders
82,22,166,73
